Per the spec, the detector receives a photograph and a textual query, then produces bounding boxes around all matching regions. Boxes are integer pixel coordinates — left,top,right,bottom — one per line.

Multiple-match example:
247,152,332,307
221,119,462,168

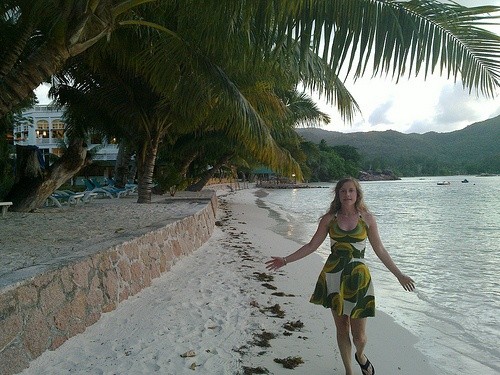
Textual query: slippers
355,352,375,375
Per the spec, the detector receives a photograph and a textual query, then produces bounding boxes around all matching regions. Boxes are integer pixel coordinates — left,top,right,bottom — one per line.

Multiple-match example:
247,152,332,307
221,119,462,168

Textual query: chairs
45,177,138,208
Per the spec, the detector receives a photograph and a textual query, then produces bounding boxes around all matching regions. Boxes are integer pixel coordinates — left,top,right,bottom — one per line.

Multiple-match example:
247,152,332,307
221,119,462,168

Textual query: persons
265,177,417,375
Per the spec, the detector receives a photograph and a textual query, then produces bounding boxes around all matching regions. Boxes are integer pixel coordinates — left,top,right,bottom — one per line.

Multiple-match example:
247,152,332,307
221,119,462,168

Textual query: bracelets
283,257,287,265
